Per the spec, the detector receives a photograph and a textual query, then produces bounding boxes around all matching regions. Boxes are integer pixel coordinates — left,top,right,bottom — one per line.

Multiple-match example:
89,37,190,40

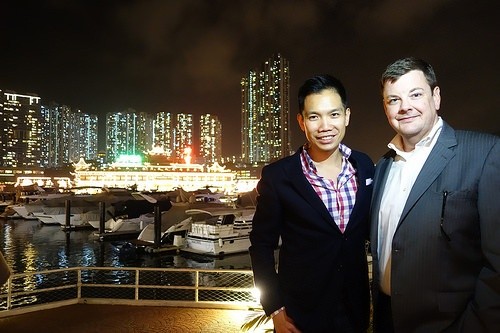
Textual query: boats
6,188,233,247
187,206,282,255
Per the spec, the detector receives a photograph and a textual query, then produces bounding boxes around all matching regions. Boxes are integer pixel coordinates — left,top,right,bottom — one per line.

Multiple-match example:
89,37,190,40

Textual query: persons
249,74,379,333
368,55,500,333
